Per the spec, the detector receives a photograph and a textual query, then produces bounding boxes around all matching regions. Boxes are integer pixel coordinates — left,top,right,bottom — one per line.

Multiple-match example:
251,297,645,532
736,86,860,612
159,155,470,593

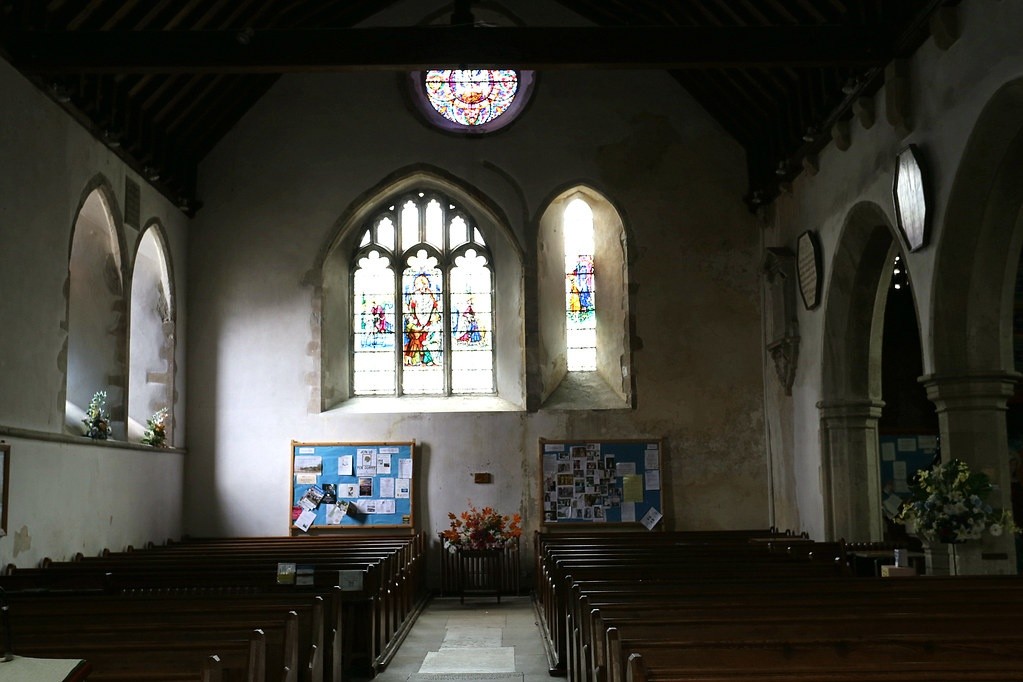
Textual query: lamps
842,67,858,94
752,191,766,203
775,161,792,173
802,125,822,142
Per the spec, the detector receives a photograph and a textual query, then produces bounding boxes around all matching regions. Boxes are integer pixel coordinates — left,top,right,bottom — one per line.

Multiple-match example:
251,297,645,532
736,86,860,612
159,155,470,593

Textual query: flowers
894,459,1023,542
441,499,522,553
82,390,112,440
142,408,173,448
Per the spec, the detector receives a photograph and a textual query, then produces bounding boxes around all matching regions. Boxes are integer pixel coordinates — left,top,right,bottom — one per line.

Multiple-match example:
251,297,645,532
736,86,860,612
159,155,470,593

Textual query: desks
846,549,925,577
460,549,502,603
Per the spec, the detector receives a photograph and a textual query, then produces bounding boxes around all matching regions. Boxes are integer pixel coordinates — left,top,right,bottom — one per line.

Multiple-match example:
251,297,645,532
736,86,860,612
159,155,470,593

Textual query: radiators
438,532,520,597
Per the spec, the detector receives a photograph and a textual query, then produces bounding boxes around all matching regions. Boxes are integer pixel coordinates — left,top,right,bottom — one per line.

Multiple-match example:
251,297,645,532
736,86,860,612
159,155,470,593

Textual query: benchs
0,534,433,682
533,529,1023,682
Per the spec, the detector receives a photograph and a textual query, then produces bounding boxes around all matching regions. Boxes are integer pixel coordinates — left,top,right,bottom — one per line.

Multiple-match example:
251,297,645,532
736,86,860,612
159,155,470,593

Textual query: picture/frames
0,445,11,537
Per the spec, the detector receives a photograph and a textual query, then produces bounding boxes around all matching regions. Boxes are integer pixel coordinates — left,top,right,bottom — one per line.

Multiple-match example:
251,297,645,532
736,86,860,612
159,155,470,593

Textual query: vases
921,543,951,576
